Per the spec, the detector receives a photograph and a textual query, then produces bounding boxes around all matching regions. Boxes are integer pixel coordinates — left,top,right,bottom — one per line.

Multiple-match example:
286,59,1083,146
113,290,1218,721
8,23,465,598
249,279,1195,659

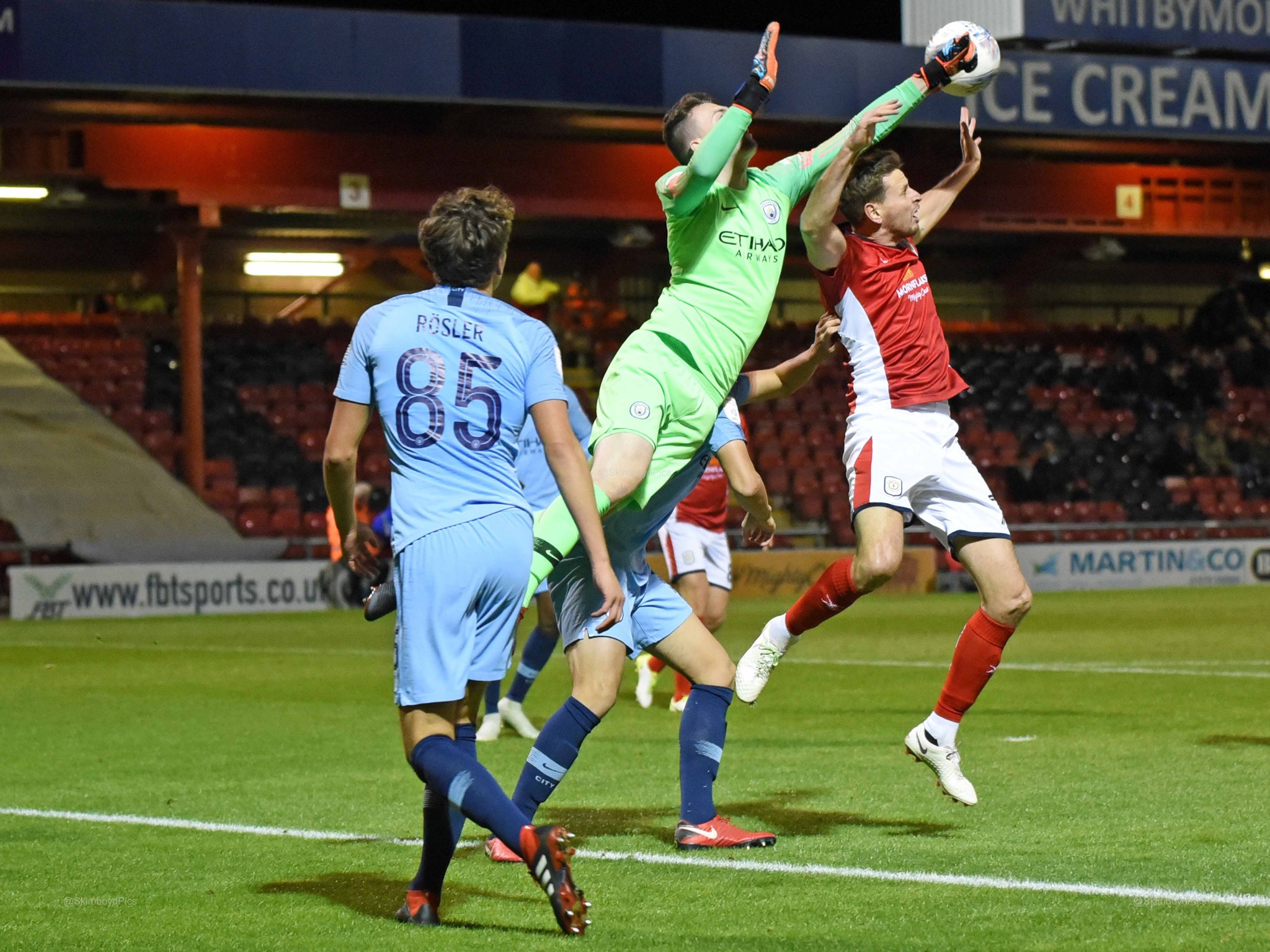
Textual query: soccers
925,20,1004,99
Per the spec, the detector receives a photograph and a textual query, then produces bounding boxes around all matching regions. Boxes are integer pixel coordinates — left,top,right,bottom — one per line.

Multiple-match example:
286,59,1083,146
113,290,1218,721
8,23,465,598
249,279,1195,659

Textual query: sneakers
524,822,592,937
904,721,977,807
674,814,777,850
363,579,398,621
498,695,541,740
735,617,786,703
634,653,659,709
668,693,688,713
484,837,523,862
474,713,503,741
393,890,441,926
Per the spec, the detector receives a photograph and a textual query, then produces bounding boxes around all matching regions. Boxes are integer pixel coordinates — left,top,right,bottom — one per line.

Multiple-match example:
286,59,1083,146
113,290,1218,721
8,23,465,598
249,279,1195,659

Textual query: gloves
750,20,780,92
908,31,978,90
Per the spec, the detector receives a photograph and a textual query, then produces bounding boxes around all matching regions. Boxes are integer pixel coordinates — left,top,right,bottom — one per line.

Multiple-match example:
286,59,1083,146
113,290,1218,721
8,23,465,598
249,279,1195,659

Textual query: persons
484,310,844,866
513,19,984,627
513,259,562,307
735,98,1037,807
971,318,1270,520
318,175,624,939
466,310,749,748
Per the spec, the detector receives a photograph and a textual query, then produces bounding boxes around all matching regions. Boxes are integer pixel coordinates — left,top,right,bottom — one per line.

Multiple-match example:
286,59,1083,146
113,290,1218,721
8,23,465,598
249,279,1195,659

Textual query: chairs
0,311,1269,559
